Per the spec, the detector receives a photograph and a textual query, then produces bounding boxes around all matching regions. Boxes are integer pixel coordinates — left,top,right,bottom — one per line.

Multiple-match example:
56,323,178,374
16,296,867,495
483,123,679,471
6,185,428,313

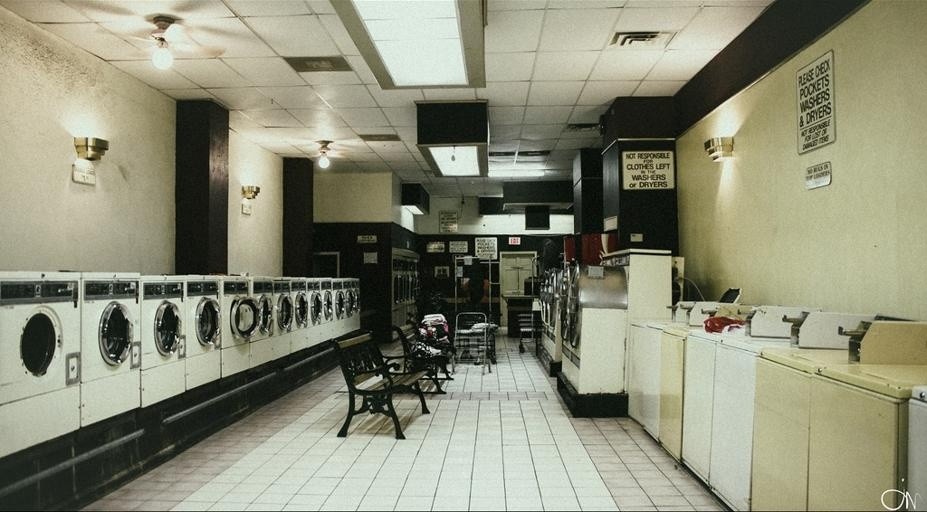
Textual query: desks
501,293,539,337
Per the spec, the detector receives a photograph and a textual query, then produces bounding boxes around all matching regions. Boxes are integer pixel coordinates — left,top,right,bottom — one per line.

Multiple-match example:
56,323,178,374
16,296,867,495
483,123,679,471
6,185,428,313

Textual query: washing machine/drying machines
391,247,422,330
535,249,926,511
0,269,362,511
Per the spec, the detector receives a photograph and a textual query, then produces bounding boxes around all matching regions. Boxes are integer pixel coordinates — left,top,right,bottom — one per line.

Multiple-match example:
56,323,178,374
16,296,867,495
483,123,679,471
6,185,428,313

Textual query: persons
463,265,489,310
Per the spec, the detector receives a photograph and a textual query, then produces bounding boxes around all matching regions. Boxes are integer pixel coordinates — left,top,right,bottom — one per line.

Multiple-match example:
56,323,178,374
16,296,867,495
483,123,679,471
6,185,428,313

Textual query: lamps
413,100,490,177
151,16,175,70
315,141,333,169
71,137,109,186
241,186,260,215
401,183,430,215
704,136,734,162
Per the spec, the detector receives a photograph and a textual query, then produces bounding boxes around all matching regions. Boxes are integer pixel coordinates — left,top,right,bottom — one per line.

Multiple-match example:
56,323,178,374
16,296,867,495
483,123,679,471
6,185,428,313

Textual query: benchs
334,312,457,439
